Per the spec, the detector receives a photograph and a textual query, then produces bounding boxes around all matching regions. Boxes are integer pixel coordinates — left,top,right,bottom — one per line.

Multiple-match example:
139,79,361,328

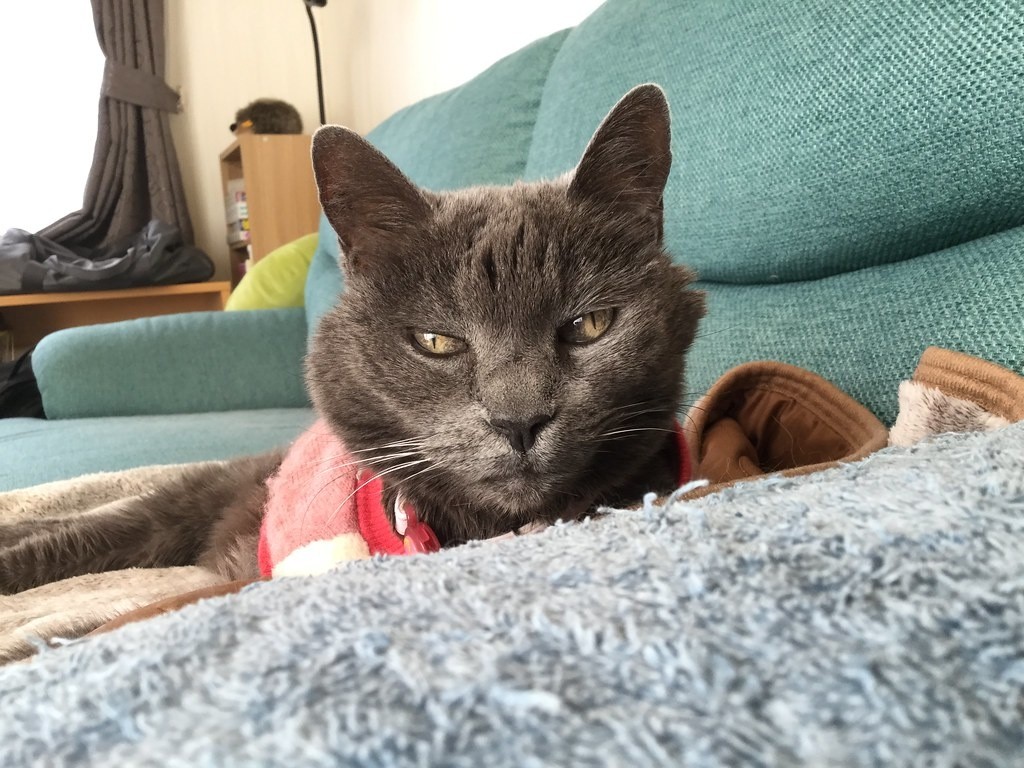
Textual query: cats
1,82,712,600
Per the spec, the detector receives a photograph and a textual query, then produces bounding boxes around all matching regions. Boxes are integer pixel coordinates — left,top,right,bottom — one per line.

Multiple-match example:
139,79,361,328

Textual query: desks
1,280,232,365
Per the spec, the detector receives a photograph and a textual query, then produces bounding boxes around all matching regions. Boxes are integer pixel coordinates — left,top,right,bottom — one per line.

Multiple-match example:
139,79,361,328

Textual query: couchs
0,1,1024,492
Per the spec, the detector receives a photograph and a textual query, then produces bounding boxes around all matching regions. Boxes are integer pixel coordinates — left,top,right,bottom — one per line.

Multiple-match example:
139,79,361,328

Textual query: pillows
222,231,318,308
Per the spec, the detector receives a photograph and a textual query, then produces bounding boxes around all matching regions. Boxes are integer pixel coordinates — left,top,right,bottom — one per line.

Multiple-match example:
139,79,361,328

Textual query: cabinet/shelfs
219,133,321,292
